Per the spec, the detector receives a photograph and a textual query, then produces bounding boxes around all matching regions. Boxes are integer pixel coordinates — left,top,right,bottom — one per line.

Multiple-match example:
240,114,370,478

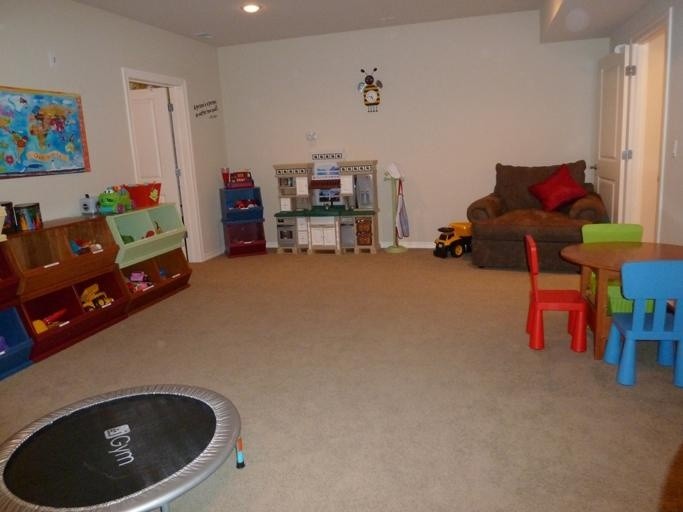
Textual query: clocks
357,68,383,112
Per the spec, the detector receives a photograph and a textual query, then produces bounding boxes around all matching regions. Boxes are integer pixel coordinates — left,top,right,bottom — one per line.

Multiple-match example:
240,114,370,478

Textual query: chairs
526,224,683,389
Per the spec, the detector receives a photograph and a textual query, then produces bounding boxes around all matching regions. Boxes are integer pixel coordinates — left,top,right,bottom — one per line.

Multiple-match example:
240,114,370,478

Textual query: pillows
494,160,586,213
528,163,589,213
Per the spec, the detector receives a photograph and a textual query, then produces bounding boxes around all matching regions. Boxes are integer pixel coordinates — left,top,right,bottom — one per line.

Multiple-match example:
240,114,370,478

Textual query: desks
0,383,245,512
272,160,381,255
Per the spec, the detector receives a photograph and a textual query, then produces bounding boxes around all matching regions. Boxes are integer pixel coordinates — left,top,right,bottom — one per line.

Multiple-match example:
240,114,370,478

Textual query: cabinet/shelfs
219,187,268,259
0,202,192,382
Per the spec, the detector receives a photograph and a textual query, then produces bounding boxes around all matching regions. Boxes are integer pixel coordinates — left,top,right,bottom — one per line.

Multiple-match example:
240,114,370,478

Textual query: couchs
467,183,606,273
